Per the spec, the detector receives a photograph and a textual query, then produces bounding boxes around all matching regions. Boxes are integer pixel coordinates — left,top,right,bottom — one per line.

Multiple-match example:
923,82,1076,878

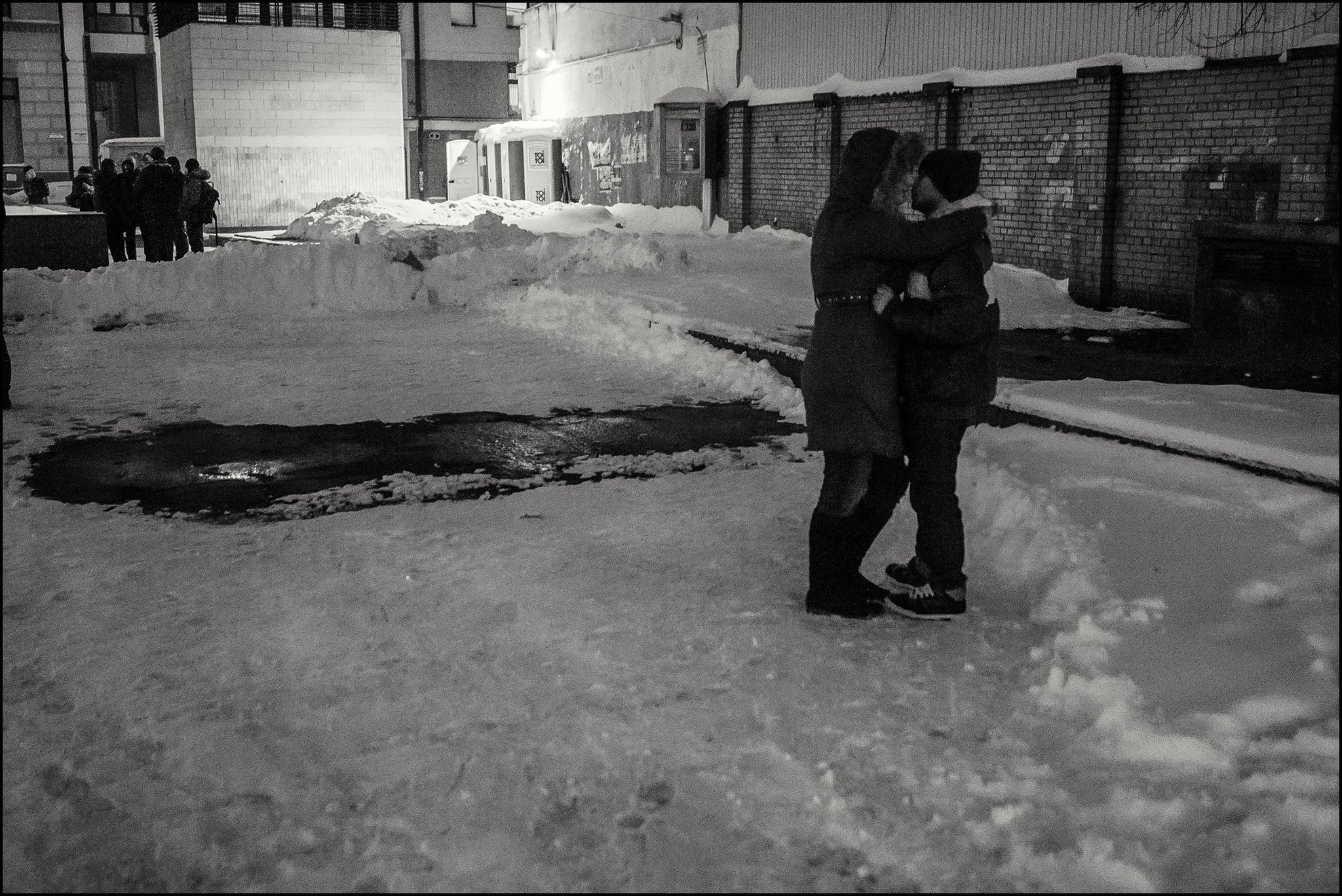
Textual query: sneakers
883,572,968,619
885,556,932,589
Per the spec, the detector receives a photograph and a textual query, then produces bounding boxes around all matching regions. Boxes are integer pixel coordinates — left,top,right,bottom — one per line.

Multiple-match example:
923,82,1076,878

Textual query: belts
818,290,874,307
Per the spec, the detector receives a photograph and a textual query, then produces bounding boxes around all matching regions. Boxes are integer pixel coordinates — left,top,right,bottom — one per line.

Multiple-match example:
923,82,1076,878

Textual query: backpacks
193,174,219,224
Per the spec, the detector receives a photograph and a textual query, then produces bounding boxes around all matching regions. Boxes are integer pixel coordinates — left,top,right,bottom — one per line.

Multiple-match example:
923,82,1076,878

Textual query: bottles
1255,196,1266,222
354,233,360,245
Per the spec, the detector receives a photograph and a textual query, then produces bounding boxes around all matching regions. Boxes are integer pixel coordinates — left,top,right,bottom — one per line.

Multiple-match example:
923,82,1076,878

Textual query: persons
803,125,1002,618
871,140,1004,620
64,145,221,263
21,166,51,206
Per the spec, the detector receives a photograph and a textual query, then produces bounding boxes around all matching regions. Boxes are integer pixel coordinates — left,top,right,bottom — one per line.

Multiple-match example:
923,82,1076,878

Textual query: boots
851,497,896,599
806,508,883,619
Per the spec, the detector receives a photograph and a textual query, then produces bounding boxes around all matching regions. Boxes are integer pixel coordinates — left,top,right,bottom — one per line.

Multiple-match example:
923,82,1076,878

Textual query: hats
149,146,165,160
185,158,199,171
919,148,981,203
